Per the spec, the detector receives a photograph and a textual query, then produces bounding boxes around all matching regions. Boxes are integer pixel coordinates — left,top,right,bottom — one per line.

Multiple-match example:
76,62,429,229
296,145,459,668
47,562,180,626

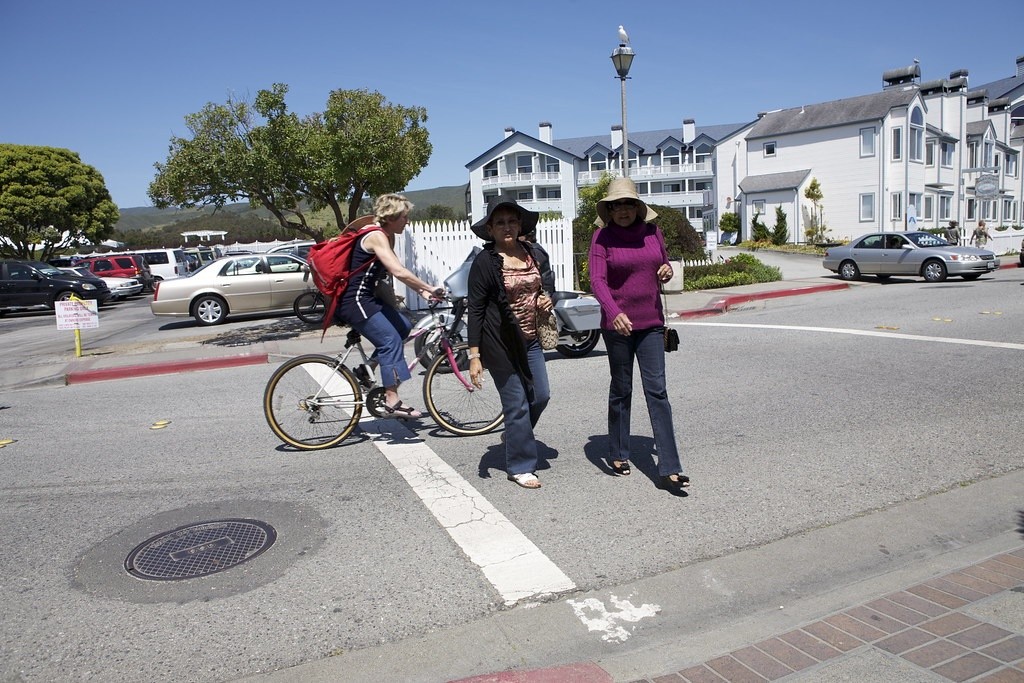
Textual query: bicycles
293,283,349,327
263,287,506,451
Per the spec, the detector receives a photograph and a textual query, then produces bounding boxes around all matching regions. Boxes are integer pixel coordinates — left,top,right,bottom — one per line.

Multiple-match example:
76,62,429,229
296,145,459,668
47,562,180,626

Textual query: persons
588,177,691,488
467,195,560,489
887,238,900,249
944,220,961,246
970,220,993,250
334,193,449,418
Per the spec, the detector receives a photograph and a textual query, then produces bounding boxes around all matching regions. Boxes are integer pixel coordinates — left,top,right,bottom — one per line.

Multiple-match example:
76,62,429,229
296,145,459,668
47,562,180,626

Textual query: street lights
611,25,635,176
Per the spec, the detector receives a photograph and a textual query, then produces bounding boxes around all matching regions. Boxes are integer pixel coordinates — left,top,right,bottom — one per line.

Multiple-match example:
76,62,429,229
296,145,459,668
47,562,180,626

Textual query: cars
28,266,144,302
149,251,325,327
823,230,1001,283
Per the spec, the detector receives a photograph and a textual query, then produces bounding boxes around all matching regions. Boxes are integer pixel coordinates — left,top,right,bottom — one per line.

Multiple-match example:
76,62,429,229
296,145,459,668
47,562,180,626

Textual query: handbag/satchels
521,241,559,350
664,326,680,352
374,275,395,307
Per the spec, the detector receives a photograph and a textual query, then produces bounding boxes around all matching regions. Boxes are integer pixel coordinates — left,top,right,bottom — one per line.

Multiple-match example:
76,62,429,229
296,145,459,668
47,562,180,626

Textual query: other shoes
501,432,506,445
508,473,541,488
659,473,690,487
610,458,631,475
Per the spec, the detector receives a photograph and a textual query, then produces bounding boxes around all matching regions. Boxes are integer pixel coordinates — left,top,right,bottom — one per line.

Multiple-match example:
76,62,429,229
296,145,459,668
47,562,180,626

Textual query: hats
470,195,539,241
596,178,647,226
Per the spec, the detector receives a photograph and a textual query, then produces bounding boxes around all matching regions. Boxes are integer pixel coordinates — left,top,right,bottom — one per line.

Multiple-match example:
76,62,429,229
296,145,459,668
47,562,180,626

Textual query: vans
72,254,156,294
104,242,242,285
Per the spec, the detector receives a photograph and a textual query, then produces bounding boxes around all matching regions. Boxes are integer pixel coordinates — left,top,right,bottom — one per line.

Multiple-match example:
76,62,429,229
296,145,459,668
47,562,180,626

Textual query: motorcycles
408,246,603,375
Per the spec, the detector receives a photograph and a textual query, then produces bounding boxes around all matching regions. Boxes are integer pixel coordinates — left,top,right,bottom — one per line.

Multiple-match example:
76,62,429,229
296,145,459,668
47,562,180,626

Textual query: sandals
382,401,423,418
353,364,375,388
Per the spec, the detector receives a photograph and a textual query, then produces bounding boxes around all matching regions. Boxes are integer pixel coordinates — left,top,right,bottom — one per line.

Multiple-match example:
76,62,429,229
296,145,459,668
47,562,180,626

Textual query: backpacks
306,226,389,343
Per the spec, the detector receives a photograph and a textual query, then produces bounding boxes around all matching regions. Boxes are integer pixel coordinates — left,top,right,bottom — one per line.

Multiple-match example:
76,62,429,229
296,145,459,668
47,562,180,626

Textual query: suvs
0,259,112,313
226,239,319,272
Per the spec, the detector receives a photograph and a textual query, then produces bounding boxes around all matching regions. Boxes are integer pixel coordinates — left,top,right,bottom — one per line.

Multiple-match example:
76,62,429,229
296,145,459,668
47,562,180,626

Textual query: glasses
492,217,520,229
609,200,639,212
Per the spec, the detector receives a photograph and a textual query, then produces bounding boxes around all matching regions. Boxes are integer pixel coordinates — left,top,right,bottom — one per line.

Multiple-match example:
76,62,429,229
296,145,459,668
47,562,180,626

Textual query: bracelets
418,289,424,296
467,353,480,361
